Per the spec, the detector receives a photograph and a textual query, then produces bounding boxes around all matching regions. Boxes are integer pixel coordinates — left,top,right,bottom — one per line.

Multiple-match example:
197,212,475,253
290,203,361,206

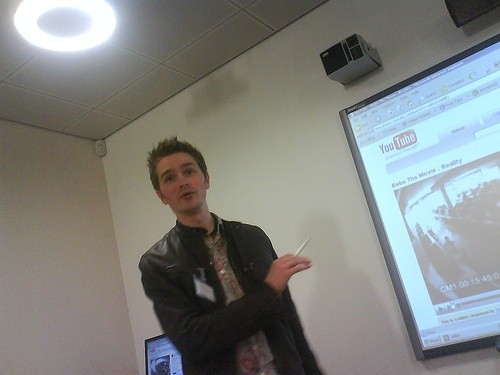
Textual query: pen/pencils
292,236,312,256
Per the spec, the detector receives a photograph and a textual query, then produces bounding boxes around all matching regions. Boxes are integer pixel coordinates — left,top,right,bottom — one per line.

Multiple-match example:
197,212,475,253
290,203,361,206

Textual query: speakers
320,34,381,87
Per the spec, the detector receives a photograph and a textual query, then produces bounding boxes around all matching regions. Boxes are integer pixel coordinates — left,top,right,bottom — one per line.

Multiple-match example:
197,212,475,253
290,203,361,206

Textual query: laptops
145,331,183,375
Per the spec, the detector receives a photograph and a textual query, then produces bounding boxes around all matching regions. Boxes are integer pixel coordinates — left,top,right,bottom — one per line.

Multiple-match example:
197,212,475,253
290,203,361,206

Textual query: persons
138,136,323,375
